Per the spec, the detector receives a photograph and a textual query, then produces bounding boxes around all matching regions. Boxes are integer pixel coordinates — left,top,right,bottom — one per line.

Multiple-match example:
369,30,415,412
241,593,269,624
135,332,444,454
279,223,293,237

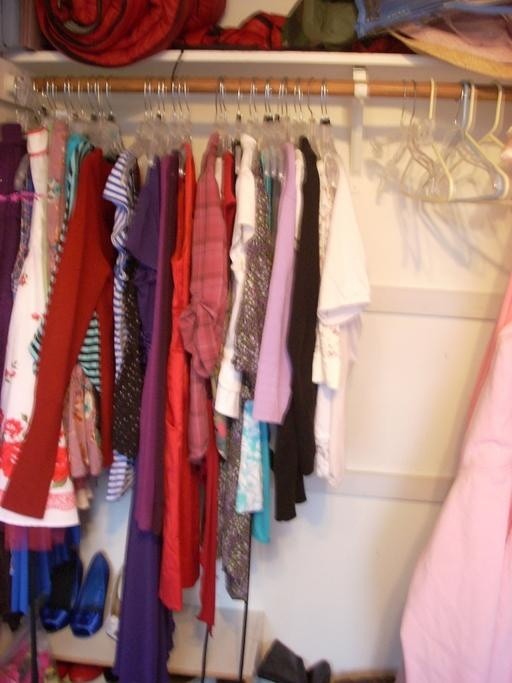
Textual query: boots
306,659,330,682
258,640,310,683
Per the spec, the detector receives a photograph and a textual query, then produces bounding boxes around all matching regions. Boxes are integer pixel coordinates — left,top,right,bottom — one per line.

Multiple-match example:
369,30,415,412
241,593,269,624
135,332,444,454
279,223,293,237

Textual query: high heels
104,563,125,641
39,551,83,632
70,551,110,636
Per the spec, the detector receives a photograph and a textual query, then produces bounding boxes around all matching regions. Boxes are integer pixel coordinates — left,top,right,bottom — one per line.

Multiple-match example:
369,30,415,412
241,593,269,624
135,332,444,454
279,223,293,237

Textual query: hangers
373,76,512,212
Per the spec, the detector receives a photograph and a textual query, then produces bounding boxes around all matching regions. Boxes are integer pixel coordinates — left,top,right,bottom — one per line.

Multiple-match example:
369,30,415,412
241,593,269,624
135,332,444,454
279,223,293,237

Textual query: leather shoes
56,661,69,679
68,664,105,682
